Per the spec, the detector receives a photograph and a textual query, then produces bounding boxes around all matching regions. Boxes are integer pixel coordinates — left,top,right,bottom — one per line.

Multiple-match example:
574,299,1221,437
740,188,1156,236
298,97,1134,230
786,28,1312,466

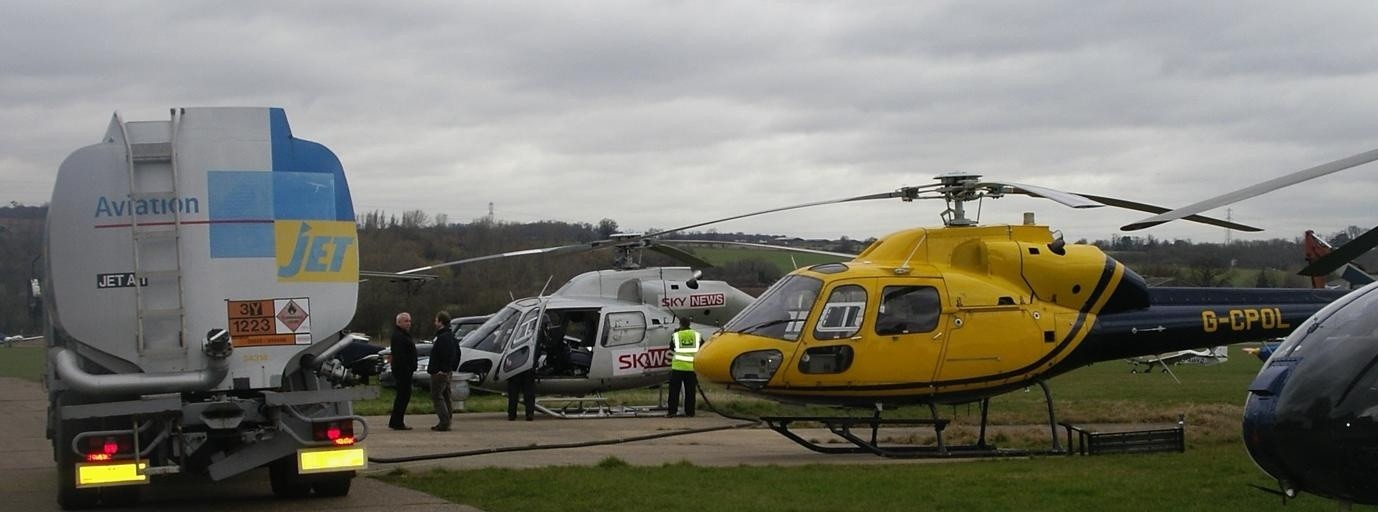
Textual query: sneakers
666,411,677,418
430,424,452,431
526,414,535,422
388,424,413,431
508,414,517,422
686,412,696,418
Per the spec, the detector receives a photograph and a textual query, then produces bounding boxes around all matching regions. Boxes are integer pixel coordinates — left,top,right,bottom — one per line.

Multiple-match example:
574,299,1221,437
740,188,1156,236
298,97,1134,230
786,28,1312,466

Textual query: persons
666,315,705,417
427,311,460,431
543,312,588,376
387,312,418,431
506,330,539,422
875,290,907,335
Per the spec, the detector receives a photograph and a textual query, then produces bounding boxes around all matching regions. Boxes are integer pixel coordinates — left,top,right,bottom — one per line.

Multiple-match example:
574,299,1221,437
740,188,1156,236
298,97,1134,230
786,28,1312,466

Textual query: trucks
27,107,380,509
